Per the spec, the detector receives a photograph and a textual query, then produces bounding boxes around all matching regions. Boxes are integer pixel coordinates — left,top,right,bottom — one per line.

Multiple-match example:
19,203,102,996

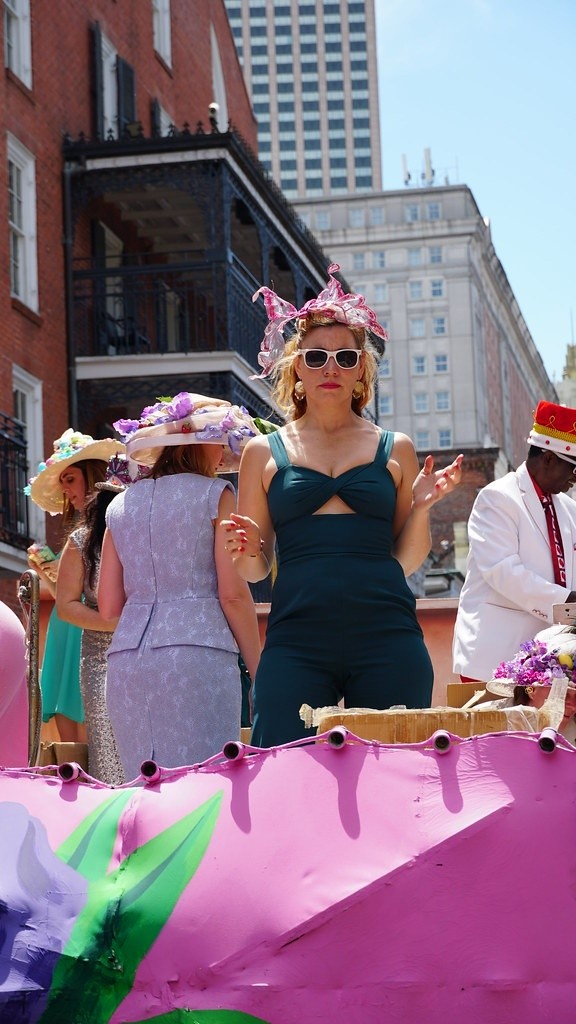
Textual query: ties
542,496,567,588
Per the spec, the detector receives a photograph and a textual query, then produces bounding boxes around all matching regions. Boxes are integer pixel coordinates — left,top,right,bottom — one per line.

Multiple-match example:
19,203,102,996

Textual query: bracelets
249,553,261,557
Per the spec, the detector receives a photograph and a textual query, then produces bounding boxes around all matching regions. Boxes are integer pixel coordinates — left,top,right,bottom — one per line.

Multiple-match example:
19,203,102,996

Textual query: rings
224,545,228,552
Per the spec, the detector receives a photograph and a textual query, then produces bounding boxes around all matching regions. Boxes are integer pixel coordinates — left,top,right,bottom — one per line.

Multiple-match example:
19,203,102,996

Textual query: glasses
542,449,576,475
297,348,362,369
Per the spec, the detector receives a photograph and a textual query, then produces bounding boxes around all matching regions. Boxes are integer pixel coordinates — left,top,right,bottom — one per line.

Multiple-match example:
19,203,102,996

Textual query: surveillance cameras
207,103,220,118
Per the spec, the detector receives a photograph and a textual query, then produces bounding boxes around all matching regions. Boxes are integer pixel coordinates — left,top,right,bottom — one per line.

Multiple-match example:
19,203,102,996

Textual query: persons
219,264,464,755
452,400,576,683
22,427,127,741
56,454,127,787
96,390,261,781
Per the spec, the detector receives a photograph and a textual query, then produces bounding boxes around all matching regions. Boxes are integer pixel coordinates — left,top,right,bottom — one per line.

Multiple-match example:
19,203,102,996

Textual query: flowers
112,392,195,443
494,639,573,686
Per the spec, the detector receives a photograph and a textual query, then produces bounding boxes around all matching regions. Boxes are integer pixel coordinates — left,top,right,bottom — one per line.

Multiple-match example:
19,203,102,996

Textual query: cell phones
29,546,55,565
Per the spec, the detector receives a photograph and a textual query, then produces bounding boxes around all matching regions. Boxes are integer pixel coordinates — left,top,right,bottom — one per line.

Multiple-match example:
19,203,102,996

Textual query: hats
113,392,264,472
25,429,127,516
527,400,576,456
